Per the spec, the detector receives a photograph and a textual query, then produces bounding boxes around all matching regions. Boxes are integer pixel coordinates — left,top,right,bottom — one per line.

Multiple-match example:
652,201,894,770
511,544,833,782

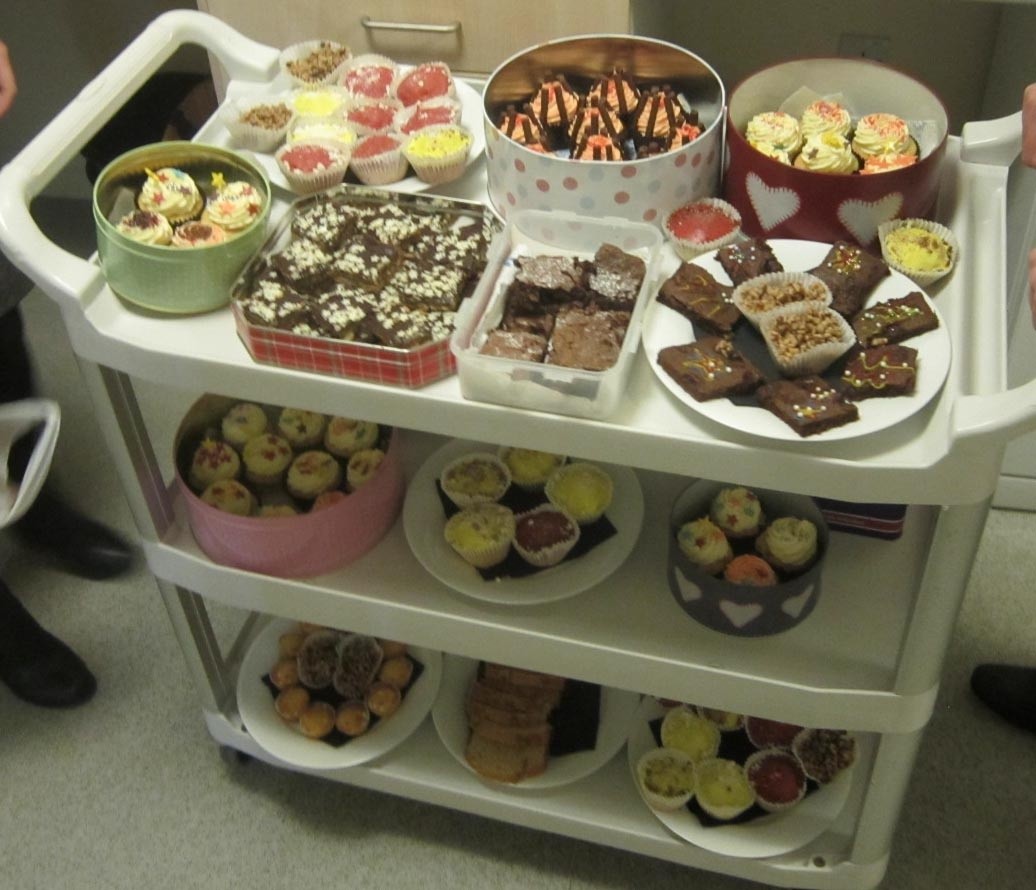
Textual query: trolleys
0,5,1036,890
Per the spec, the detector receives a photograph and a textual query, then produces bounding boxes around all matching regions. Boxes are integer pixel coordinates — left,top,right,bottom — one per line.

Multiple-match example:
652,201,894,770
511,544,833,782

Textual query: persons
0,44,134,709
971,83,1036,738
69,0,218,202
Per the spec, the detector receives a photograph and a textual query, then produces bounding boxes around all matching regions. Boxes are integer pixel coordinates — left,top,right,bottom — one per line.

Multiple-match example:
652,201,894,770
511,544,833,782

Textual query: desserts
678,487,817,584
219,38,473,192
636,704,860,819
269,621,414,737
878,218,957,287
746,98,918,174
113,167,262,248
662,198,739,262
497,66,705,163
192,403,384,518
442,445,613,566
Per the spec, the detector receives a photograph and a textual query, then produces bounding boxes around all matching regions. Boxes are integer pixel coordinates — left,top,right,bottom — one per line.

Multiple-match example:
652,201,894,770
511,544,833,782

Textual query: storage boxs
451,209,663,419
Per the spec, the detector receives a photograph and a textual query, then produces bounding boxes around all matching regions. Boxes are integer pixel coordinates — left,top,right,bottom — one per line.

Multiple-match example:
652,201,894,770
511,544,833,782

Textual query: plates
431,652,640,789
238,617,441,769
253,63,486,196
642,239,951,442
629,696,855,858
403,436,645,604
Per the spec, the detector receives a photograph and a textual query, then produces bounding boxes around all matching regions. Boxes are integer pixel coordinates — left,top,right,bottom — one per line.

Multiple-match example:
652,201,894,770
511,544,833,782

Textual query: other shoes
2,593,96,708
20,514,132,580
972,665,1036,738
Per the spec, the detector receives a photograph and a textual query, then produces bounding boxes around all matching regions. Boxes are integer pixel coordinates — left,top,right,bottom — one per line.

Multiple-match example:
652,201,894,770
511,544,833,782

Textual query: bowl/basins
723,56,948,261
449,208,663,420
93,140,271,313
482,33,725,253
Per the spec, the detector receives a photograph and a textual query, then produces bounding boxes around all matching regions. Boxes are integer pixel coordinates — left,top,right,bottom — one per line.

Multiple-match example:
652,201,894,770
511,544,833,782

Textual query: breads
480,244,647,405
466,665,564,781
656,238,940,438
242,198,490,349
